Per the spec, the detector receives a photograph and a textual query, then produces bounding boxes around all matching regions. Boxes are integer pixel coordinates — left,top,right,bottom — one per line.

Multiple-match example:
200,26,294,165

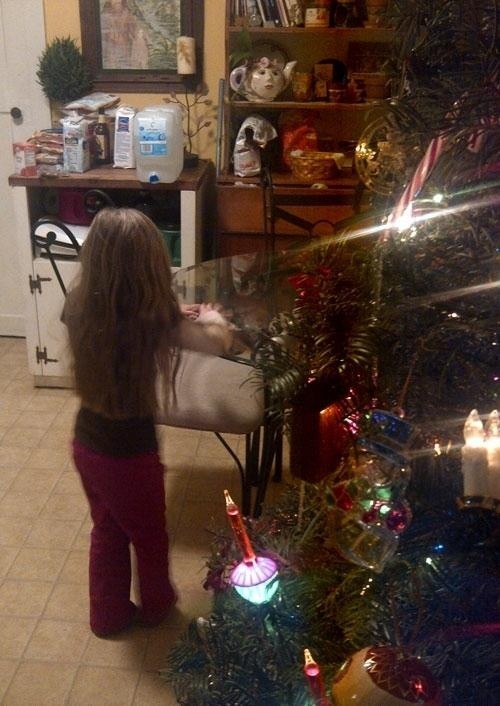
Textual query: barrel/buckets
135,105,185,184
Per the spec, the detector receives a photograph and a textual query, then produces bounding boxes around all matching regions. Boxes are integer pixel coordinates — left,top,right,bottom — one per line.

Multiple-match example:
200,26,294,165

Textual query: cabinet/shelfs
8,159,213,389
212,0,400,260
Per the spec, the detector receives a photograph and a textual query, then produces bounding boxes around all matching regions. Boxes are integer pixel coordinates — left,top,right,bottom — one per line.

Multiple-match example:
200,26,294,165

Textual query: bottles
233,125,267,176
94,105,110,162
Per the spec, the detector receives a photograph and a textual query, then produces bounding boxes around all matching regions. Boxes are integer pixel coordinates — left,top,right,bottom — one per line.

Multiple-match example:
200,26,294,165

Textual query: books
230,0,305,27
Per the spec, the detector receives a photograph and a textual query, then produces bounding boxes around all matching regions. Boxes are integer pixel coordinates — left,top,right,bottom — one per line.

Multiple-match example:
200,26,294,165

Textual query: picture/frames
78,0,204,94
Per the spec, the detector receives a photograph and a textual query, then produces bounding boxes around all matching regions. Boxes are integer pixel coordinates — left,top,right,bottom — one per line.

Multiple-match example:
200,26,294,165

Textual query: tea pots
226,56,297,101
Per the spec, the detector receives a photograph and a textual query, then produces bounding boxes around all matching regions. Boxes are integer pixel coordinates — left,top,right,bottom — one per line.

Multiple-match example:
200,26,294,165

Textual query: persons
58,204,235,639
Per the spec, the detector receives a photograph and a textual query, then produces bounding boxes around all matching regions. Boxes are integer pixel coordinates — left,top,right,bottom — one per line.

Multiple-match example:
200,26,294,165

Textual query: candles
462,409,500,500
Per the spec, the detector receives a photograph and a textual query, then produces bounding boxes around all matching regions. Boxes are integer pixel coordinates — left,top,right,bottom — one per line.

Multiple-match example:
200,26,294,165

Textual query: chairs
261,167,366,309
30,188,282,523
